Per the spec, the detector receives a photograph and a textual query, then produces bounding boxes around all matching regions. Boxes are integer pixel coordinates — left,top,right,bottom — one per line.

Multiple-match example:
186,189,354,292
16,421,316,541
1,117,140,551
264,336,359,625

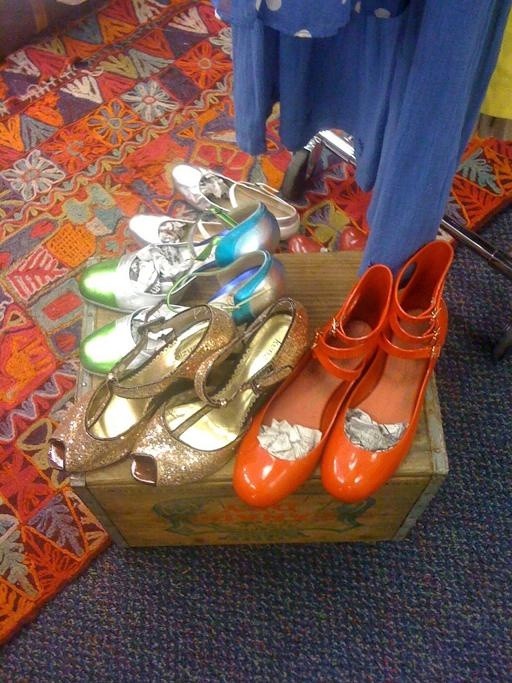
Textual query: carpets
0,1,512,646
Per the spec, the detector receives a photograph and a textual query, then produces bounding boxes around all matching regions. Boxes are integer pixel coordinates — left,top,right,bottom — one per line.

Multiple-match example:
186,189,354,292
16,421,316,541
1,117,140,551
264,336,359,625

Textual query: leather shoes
76,201,287,314
322,239,455,503
75,248,283,382
169,158,305,241
127,204,279,257
232,263,393,509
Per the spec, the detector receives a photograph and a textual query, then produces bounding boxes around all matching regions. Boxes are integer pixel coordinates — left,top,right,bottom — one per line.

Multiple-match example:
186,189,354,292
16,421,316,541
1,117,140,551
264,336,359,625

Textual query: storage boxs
71,251,451,548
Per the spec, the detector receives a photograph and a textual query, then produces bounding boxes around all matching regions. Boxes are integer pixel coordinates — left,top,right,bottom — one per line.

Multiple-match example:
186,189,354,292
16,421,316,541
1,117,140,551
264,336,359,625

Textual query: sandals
48,301,236,475
129,297,310,490
